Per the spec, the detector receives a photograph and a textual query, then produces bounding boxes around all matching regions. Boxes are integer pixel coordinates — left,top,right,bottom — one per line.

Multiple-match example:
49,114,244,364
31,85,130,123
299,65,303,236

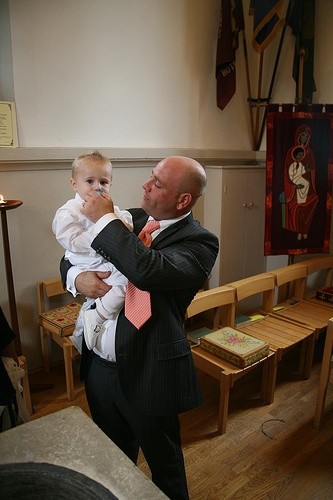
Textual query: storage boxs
199,327,269,368
35,302,83,337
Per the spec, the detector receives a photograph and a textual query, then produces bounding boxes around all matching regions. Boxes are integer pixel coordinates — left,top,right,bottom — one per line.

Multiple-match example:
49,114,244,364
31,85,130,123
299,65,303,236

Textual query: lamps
0,195,55,393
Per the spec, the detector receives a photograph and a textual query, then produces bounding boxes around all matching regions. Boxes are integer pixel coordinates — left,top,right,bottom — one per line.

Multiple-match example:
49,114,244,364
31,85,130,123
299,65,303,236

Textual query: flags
215,0,245,111
248,0,284,53
263,105,333,256
286,0,317,106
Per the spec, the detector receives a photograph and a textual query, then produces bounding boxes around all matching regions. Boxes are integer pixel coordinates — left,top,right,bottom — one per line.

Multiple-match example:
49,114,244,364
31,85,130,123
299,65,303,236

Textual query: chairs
184,286,278,436
302,256,333,364
34,277,83,401
269,263,333,380
226,272,316,401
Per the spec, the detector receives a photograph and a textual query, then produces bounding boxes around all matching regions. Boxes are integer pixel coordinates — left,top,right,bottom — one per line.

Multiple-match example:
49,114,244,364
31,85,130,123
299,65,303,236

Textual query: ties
125,220,161,330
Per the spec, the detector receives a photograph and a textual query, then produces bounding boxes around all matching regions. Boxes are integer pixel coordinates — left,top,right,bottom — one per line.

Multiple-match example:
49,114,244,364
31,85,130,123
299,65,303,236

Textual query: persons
51,151,133,350
59,156,220,500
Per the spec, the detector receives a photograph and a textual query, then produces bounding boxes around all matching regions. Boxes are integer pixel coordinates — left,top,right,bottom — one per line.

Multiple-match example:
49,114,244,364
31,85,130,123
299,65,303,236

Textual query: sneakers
83,309,104,350
69,336,81,355
